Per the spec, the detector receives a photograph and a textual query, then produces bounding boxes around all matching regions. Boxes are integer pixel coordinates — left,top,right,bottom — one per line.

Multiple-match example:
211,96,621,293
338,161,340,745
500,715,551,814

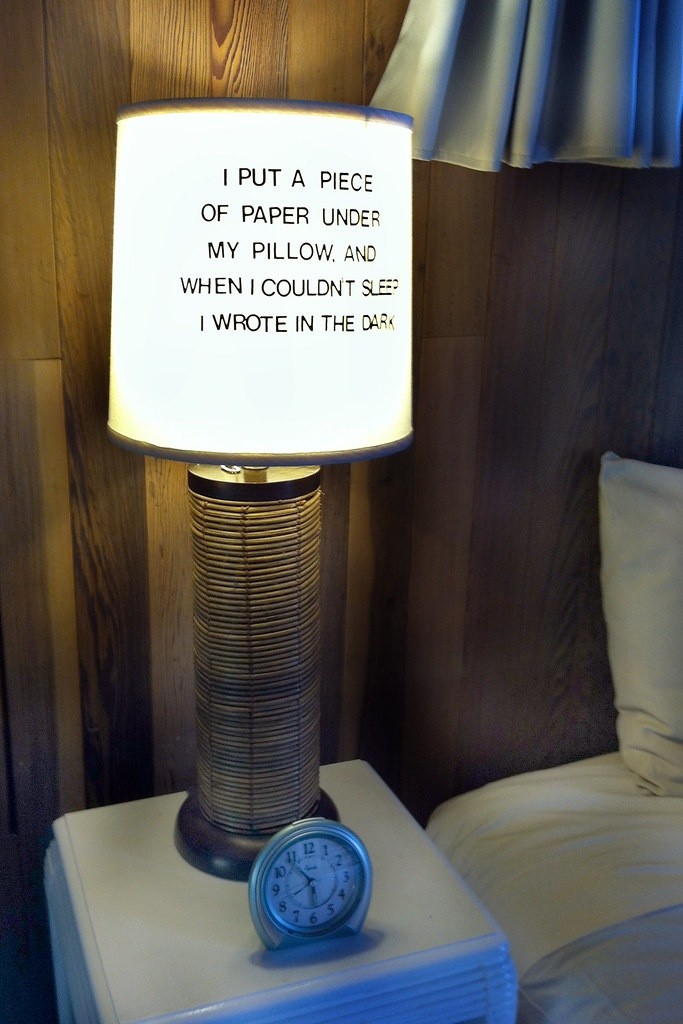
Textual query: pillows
600,450,682,800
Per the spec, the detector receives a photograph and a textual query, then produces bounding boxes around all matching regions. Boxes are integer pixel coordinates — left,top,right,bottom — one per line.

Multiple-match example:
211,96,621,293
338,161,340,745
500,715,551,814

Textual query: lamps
102,99,416,885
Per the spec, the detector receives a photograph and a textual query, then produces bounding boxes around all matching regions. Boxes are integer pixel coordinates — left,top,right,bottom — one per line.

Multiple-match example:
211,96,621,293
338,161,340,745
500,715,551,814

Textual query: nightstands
43,760,519,1024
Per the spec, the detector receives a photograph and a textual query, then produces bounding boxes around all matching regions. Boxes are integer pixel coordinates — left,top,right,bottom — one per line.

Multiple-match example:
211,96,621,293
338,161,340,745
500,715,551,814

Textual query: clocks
247,816,374,950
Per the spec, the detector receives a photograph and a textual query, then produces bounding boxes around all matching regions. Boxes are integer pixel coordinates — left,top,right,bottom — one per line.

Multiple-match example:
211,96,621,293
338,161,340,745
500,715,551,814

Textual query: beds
424,750,682,1024
